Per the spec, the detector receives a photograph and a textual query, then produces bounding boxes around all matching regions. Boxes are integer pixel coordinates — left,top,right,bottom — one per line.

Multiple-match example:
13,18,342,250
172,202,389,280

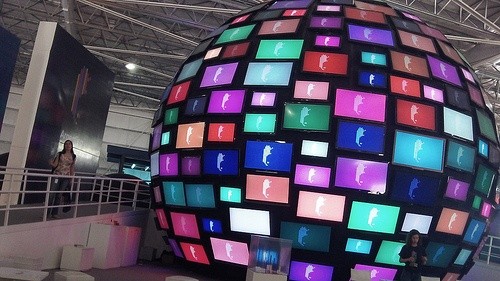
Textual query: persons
47,140,78,219
394,228,427,281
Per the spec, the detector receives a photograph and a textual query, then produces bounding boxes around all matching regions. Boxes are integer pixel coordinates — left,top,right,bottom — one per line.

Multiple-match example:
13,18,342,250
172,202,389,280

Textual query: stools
60,247,94,271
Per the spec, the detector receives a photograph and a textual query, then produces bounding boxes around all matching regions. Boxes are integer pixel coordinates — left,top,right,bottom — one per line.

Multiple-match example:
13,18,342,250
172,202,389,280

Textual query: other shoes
40,214,49,219
52,214,61,219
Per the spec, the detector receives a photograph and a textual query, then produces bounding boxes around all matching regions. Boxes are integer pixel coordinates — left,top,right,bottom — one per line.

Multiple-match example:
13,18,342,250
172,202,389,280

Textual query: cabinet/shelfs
85,223,141,269
